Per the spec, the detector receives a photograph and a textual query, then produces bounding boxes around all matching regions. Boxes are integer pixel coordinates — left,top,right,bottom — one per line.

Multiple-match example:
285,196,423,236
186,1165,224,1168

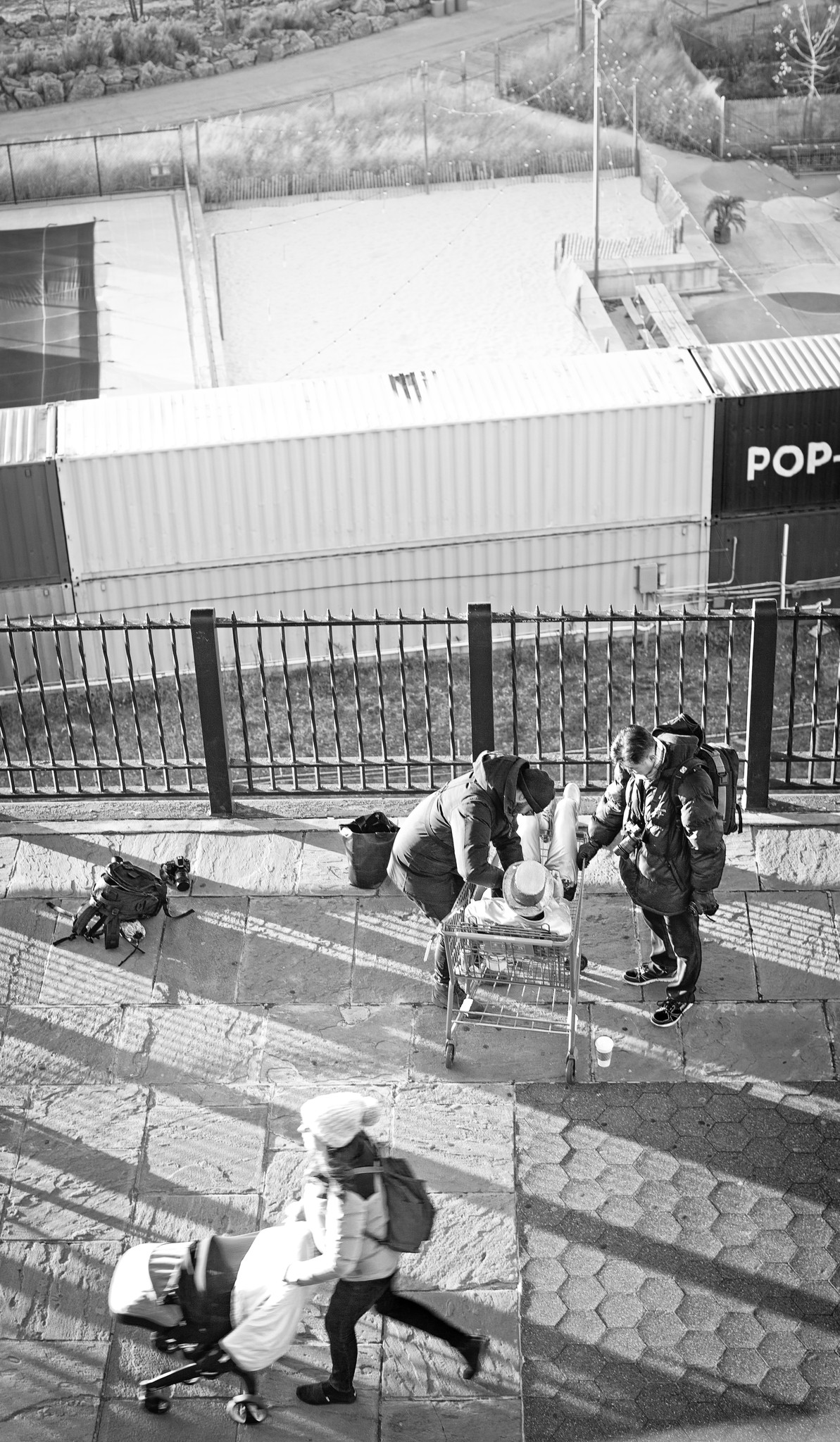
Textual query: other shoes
295,1382,357,1406
537,796,555,843
563,782,582,831
457,1334,490,1379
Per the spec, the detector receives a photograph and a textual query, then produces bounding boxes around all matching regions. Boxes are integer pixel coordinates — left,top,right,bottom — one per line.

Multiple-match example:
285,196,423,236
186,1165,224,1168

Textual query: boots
461,957,508,986
432,973,485,1019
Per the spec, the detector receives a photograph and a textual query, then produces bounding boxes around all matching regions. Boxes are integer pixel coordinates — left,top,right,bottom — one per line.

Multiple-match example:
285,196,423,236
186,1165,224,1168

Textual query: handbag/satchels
338,812,401,889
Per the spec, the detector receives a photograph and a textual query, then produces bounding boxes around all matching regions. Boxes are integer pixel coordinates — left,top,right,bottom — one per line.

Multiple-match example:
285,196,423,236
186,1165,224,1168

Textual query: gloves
692,889,719,916
576,837,602,871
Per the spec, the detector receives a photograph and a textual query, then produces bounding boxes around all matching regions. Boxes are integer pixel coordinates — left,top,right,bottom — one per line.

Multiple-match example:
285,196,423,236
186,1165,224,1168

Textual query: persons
465,783,581,956
577,714,726,1028
284,1091,490,1406
386,751,555,1019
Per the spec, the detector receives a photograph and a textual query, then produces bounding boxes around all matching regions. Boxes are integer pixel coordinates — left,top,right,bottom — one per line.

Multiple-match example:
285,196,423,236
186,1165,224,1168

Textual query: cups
595,1036,614,1067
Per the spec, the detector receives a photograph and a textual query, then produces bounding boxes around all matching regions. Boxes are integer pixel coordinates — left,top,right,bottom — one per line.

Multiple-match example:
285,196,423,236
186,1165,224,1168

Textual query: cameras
159,855,191,892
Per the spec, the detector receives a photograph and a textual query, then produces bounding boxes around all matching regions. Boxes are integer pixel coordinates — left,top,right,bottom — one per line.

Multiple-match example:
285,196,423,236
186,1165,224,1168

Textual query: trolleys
421,814,592,1088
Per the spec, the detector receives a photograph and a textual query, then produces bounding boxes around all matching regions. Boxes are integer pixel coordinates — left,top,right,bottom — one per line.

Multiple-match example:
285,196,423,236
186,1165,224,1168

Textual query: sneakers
651,993,695,1027
622,962,679,986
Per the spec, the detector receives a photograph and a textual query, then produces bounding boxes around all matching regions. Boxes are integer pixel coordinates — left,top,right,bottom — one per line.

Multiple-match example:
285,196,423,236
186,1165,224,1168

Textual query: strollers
108,1224,315,1420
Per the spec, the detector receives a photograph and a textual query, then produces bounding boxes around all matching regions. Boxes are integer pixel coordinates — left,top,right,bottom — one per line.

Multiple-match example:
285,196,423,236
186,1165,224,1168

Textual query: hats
502,860,554,917
301,1092,381,1149
518,768,556,814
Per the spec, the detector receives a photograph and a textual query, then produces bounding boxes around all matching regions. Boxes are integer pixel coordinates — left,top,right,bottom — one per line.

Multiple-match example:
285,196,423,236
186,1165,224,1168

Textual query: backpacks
672,742,742,835
344,1141,437,1253
45,855,196,966
